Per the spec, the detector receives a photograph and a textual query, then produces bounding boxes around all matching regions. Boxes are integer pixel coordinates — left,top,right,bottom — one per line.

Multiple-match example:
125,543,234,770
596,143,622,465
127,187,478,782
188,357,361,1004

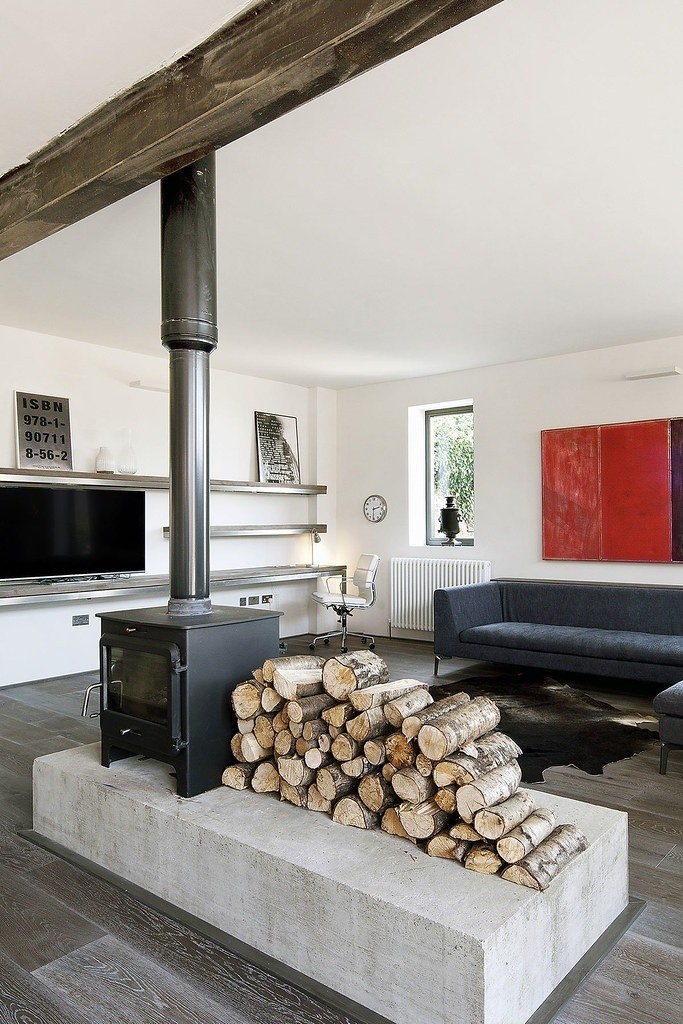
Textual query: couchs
434,577,683,774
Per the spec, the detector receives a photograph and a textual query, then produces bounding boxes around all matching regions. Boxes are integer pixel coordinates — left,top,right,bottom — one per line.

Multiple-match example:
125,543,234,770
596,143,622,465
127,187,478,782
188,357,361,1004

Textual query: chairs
308,554,381,655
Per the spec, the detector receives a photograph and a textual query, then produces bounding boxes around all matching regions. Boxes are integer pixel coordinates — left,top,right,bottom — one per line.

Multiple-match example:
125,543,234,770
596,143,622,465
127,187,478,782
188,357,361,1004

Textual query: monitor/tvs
0,481,145,582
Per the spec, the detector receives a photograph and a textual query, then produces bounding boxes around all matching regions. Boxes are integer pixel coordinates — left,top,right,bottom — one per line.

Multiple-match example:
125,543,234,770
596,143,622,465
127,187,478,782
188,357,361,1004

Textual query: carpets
427,671,661,784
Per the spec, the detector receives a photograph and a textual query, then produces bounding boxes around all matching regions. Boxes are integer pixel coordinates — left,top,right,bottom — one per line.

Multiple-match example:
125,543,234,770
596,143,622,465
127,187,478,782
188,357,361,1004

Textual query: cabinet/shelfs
0,469,328,605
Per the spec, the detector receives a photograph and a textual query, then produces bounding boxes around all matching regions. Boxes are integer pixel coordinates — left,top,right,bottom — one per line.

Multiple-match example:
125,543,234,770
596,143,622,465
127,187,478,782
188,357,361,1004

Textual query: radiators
392,556,490,631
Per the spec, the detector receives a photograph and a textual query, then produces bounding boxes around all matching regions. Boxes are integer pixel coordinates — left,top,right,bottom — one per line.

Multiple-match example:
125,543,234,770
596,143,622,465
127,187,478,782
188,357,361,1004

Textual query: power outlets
263,595,272,603
240,598,246,606
73,615,90,625
249,596,259,605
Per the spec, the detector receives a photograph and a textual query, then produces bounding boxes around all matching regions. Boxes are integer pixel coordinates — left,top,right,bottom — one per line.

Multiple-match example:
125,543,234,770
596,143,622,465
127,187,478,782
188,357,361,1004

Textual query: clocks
363,495,388,523
